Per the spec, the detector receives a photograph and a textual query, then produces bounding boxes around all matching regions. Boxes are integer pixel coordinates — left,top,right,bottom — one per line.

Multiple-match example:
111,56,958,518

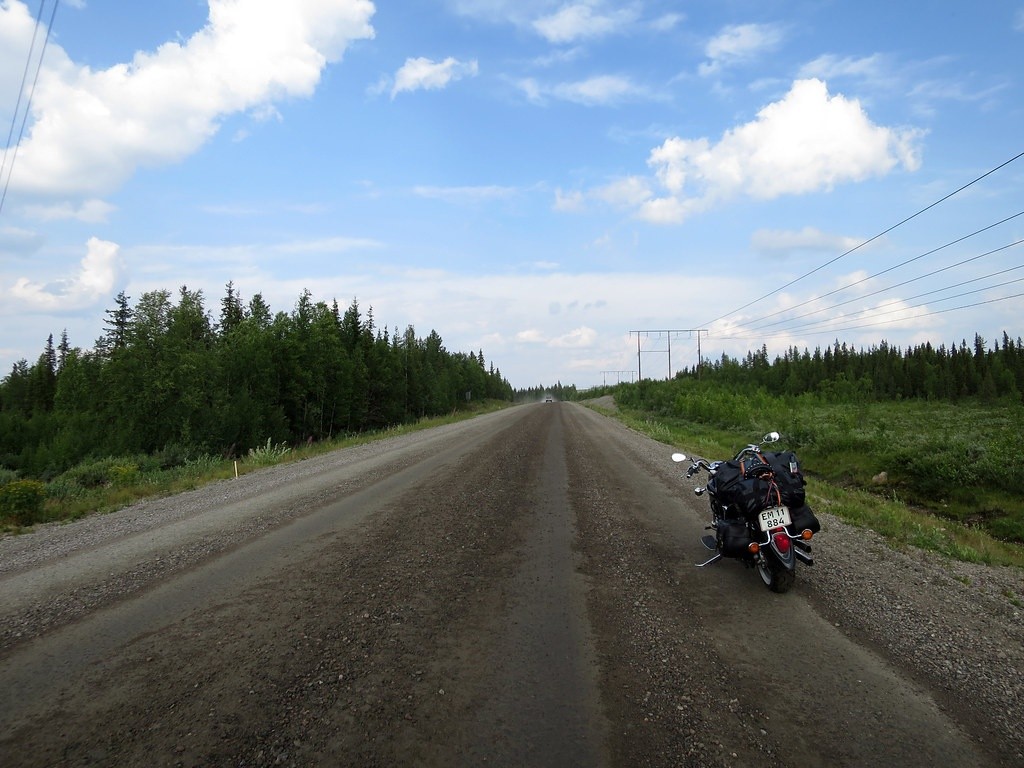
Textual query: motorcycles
670,430,816,594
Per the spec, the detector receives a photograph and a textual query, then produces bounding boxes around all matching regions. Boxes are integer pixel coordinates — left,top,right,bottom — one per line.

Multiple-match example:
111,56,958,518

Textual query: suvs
546,396,552,403
